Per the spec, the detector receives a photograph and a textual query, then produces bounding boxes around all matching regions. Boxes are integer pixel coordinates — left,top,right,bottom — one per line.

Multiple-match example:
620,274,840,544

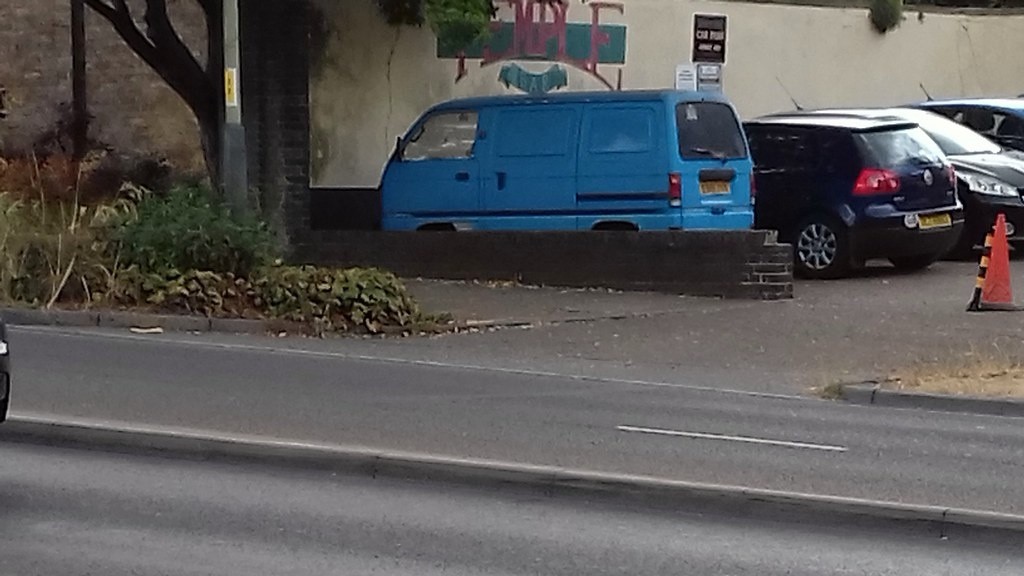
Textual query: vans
375,89,755,234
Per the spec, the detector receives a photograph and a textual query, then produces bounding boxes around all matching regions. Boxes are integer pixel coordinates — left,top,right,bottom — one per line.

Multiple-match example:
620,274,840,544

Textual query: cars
734,116,966,279
772,106,1024,249
896,97,1024,148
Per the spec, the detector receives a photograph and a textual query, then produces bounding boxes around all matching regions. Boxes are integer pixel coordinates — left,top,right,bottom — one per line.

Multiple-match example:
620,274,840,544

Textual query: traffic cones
971,214,1022,310
